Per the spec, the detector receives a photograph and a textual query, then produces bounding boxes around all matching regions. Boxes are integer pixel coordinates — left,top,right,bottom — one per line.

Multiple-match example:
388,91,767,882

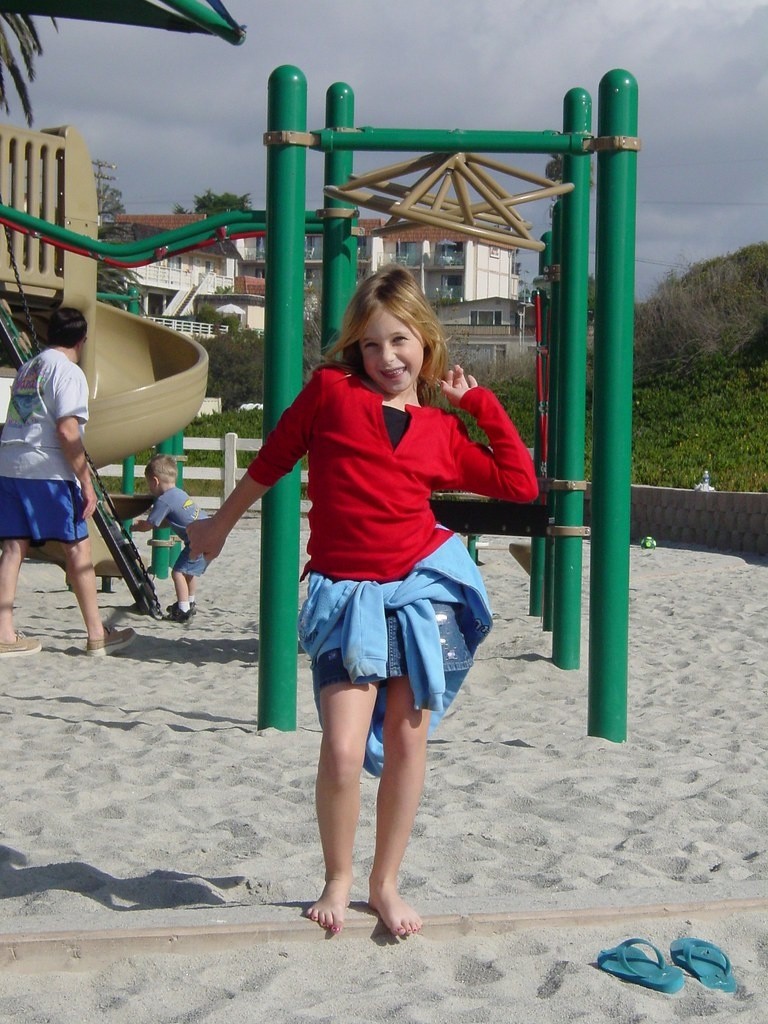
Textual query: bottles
702,471,709,491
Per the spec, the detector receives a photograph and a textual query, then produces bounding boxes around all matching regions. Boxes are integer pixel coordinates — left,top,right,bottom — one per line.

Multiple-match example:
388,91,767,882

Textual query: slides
25,300,209,579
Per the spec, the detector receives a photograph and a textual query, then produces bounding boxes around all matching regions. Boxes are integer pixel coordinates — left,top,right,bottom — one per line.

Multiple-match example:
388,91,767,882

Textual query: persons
188,265,541,937
130,454,211,622
1,304,138,657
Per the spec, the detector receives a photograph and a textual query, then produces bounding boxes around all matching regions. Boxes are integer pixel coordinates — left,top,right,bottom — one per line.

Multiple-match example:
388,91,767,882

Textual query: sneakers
162,603,198,623
0,633,42,657
87,626,136,656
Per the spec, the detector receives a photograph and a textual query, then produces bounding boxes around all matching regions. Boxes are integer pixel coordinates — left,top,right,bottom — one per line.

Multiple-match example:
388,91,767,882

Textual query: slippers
671,938,737,992
596,937,683,993
